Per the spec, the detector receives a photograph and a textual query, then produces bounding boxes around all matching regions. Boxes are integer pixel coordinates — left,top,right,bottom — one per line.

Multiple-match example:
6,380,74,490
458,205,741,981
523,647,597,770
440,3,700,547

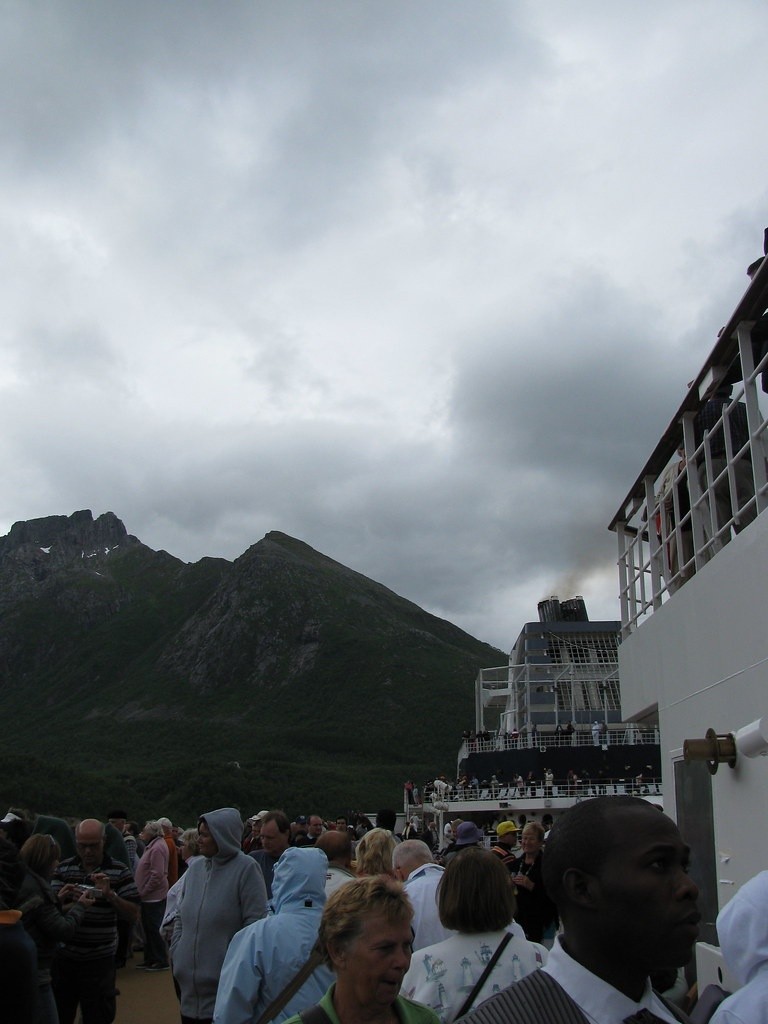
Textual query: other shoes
115,963,127,973
126,954,135,961
133,945,144,951
114,980,120,995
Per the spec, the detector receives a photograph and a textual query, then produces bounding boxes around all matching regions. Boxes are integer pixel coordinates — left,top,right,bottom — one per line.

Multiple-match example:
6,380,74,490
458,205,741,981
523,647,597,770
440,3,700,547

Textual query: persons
404,773,491,805
544,769,553,795
554,720,575,748
641,312,768,596
708,870,768,1024
454,796,702,1024
503,728,519,751
513,773,524,796
591,720,607,746
0,806,559,1024
462,729,491,752
532,723,539,748
566,770,577,794
526,771,536,795
490,775,498,787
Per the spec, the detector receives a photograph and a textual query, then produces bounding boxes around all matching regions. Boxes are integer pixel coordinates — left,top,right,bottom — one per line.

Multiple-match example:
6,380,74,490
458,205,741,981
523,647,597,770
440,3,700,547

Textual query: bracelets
107,891,118,903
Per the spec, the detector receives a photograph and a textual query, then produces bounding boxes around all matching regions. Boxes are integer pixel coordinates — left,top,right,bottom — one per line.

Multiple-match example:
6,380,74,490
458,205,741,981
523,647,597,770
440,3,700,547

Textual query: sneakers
146,962,170,971
136,961,154,969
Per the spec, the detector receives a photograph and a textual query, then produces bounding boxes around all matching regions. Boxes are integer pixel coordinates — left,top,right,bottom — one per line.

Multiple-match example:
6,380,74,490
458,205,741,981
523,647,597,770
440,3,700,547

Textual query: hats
456,822,483,845
296,816,307,824
107,810,127,819
249,811,269,821
154,817,172,828
1,813,22,823
497,821,522,837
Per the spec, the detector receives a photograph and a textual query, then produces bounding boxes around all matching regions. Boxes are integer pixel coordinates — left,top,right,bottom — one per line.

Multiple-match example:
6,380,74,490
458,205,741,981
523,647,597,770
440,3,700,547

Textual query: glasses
48,834,55,846
76,840,100,851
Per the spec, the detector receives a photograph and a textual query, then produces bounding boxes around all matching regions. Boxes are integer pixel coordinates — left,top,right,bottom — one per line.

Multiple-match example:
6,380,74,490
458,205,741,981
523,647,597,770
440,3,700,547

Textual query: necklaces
518,856,533,876
